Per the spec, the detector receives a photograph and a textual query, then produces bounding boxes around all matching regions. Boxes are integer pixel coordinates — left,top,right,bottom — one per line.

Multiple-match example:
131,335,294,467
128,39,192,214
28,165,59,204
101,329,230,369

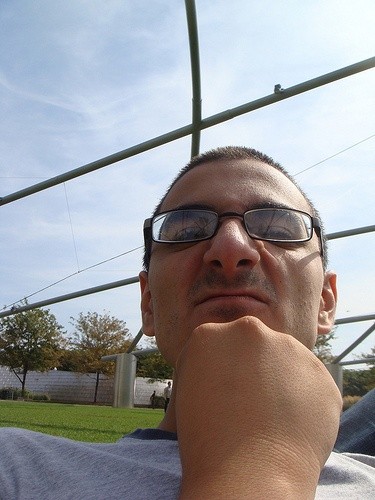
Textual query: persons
150,390,156,408
163,381,173,415
0,146,374,500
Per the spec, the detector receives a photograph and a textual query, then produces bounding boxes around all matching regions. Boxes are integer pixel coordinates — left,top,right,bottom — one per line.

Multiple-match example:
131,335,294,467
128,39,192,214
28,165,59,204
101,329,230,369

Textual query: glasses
143,206,326,269
